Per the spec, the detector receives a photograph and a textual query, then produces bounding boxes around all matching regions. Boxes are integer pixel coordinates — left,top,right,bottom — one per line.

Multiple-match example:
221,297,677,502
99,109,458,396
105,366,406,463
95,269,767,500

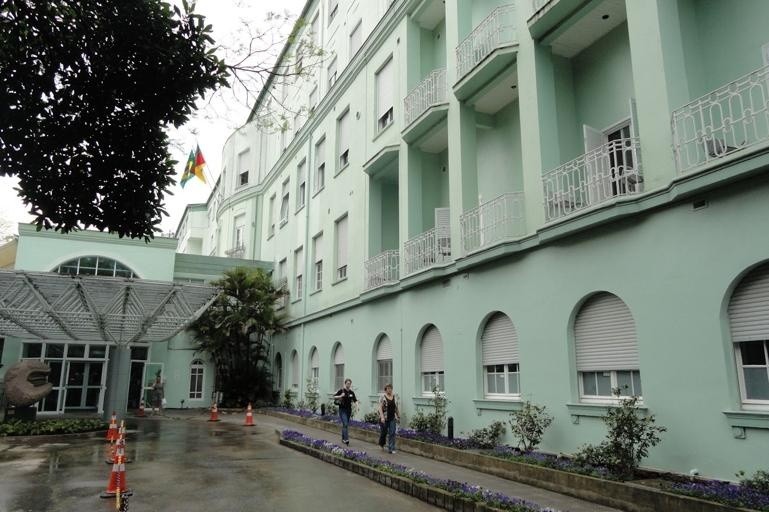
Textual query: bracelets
397,416,400,419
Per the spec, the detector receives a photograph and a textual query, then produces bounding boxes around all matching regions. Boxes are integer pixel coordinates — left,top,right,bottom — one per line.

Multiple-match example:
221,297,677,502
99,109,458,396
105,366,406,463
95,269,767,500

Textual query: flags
190,146,206,182
180,150,196,188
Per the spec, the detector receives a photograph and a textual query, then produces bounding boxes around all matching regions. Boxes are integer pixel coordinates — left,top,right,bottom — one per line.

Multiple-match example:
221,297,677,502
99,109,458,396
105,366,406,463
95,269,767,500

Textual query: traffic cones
100,410,133,498
208,402,220,422
243,401,256,426
137,397,148,418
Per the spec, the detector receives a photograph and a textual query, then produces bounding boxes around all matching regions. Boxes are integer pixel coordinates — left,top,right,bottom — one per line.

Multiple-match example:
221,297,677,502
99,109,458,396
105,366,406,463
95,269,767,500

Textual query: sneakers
378,444,396,456
340,438,349,446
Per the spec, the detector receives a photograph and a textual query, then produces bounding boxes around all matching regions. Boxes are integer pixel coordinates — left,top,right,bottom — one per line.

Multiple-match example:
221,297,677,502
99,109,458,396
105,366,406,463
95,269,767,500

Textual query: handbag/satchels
333,387,346,407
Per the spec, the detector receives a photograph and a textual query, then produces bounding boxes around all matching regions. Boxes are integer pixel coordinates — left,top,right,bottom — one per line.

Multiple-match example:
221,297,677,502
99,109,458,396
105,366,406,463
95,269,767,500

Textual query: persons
379,385,400,453
151,375,165,417
333,379,360,444
